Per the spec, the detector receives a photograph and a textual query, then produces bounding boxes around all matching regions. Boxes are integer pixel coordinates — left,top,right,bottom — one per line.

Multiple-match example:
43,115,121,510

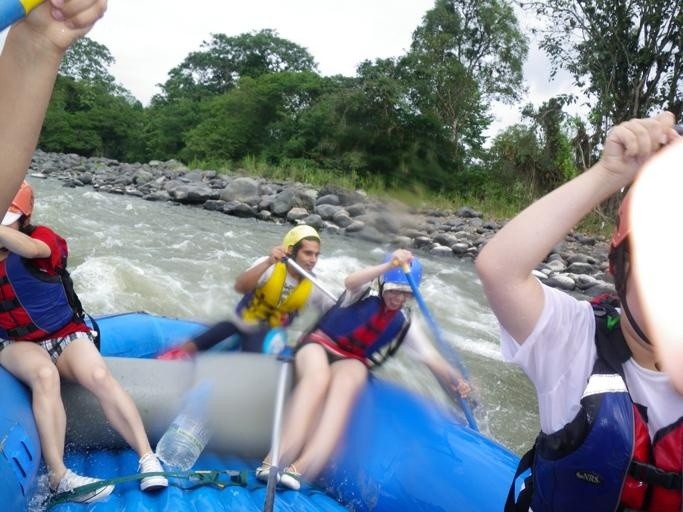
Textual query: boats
1,311,523,511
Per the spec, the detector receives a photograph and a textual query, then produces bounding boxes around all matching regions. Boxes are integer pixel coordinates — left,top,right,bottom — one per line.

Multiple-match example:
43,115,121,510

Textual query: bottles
153,402,216,474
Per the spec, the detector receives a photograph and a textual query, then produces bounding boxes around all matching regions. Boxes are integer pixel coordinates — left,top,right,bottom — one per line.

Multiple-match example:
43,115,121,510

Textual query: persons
151,223,338,375
472,104,682,512
0,1,109,226
0,182,173,505
251,246,472,494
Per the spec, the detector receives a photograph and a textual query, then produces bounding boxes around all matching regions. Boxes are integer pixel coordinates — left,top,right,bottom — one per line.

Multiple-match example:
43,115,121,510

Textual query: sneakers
139,451,168,492
47,470,115,504
280,471,302,490
256,461,280,484
156,348,190,360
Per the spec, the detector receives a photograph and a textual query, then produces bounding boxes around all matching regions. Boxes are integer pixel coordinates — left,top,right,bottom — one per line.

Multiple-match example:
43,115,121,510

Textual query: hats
1,179,34,226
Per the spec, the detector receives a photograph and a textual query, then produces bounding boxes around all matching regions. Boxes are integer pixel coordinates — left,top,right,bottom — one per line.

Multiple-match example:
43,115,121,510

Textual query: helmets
609,136,683,276
383,253,422,287
283,225,320,254
